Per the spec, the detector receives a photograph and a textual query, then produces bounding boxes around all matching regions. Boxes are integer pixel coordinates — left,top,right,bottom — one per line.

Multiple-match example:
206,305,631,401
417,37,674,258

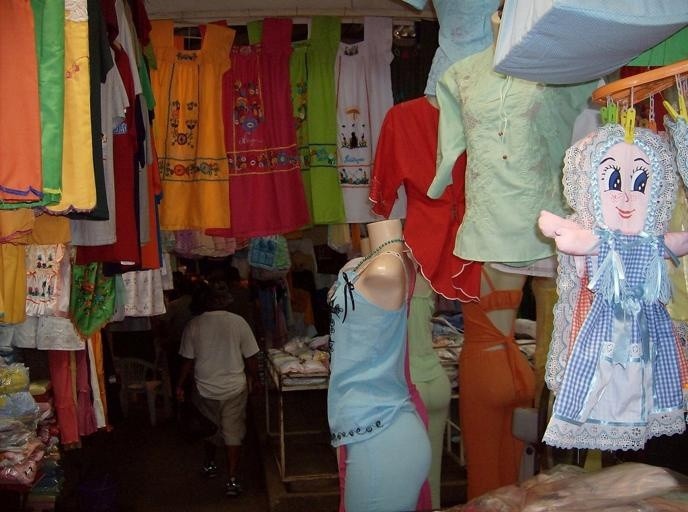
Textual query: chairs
107,328,172,427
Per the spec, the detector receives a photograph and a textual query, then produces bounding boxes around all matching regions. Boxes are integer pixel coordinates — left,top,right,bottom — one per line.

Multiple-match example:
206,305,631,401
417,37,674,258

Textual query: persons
428,10,606,406
177,279,260,497
458,262,538,503
327,220,452,510
369,95,483,303
543,125,688,450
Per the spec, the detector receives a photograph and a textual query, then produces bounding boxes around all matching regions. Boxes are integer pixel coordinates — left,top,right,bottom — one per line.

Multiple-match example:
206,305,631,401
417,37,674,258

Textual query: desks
257,348,473,483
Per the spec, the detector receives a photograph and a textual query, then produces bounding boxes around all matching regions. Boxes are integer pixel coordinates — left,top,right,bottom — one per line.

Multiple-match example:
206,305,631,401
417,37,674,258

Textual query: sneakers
226,480,241,496
201,464,217,478
